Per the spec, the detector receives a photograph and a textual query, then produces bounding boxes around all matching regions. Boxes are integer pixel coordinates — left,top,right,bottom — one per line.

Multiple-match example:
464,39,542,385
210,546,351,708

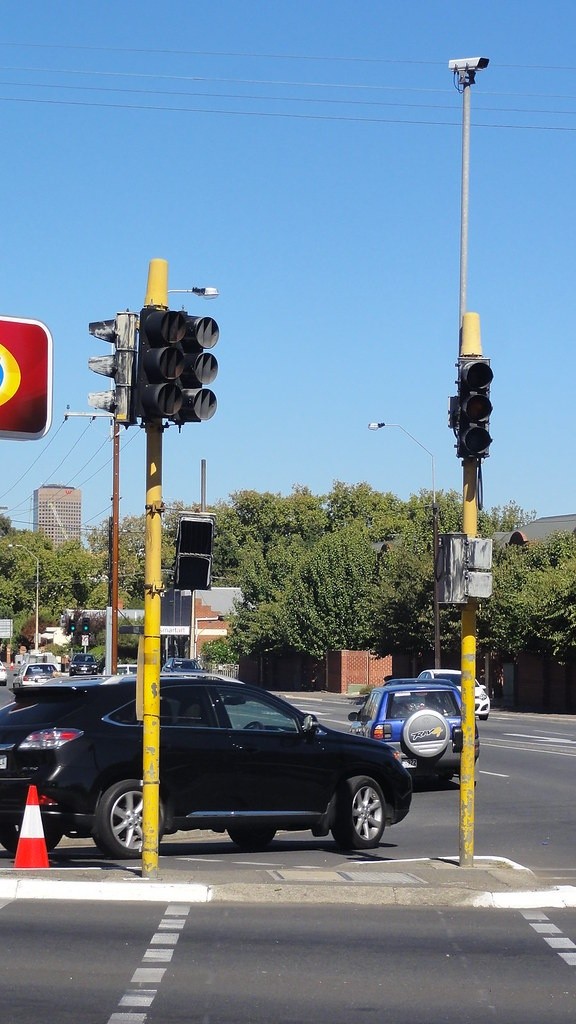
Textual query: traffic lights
131,307,188,420
69,619,76,633
452,356,495,458
179,311,220,426
173,515,213,590
88,312,136,425
82,618,90,634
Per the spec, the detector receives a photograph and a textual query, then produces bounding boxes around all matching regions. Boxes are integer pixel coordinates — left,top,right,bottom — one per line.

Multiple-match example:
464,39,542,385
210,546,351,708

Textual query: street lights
7,543,40,654
369,422,440,674
142,287,219,878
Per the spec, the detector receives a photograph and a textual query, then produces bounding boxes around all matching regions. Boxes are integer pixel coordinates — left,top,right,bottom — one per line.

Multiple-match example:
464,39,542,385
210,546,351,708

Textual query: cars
0,661,7,685
13,665,59,689
418,670,491,721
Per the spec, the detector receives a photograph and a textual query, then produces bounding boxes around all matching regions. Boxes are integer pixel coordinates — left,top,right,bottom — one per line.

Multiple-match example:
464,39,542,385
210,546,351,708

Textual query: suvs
68,653,98,676
0,678,413,859
161,657,207,679
347,681,481,787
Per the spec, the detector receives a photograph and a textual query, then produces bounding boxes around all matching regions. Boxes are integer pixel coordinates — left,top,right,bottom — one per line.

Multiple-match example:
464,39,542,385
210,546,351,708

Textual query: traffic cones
13,785,52,868
9,661,14,671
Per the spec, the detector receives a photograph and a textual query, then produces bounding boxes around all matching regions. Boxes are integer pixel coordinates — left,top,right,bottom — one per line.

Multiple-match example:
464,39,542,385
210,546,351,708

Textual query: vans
102,666,138,676
